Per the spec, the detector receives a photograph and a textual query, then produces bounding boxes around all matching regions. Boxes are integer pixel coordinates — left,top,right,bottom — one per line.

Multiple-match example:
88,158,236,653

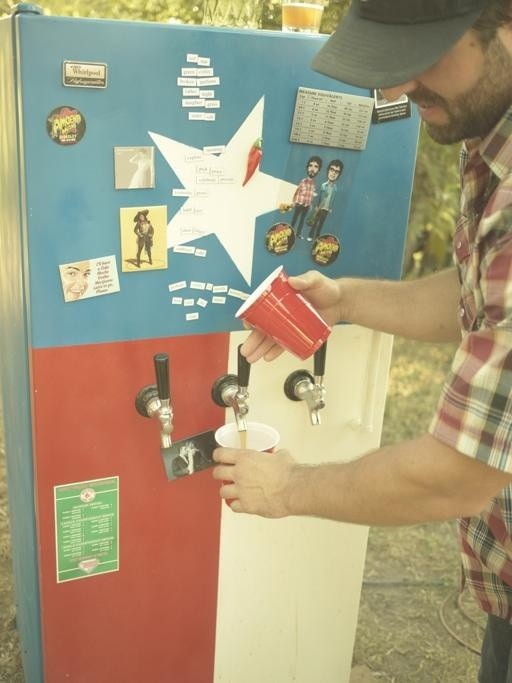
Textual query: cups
282,0,332,34
214,420,280,506
235,263,334,360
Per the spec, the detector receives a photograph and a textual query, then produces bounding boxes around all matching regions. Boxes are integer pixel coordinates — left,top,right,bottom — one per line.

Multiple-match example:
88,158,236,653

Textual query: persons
210,1,512,682
291,155,323,240
306,159,345,242
128,149,152,188
59,260,92,301
133,209,155,268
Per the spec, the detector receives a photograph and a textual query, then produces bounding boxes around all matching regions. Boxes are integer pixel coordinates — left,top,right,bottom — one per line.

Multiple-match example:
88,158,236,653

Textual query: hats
309,0,488,89
133,209,148,222
